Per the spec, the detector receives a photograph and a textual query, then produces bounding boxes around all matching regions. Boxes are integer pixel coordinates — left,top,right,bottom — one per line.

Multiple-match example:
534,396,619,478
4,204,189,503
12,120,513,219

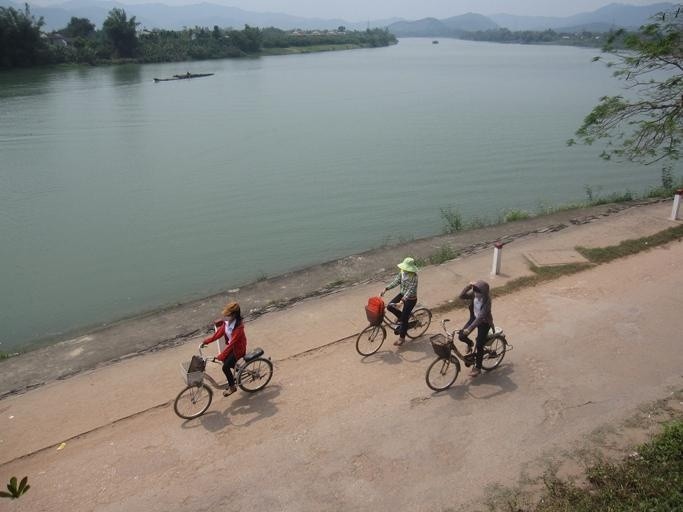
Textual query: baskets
430,334,452,357
365,306,384,323
181,363,203,385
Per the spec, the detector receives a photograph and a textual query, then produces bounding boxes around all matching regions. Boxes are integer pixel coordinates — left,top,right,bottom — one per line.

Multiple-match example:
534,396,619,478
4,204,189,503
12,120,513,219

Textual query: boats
152,70,214,83
431,40,439,44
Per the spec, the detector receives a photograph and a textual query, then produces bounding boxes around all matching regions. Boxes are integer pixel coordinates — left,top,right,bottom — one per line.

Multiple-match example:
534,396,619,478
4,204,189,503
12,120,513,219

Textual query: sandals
393,339,405,345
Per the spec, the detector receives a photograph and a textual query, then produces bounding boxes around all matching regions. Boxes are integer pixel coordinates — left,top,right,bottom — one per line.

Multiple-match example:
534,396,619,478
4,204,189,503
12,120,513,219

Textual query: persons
459,279,496,378
379,256,419,346
198,301,246,397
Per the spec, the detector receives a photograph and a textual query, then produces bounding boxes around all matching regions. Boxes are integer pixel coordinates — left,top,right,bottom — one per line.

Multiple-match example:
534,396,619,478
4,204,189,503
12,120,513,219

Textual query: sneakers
468,369,481,376
223,386,238,396
465,342,474,354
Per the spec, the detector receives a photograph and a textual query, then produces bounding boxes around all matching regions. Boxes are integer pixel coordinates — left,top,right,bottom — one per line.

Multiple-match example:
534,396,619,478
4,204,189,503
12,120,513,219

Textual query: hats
222,303,240,316
397,257,418,272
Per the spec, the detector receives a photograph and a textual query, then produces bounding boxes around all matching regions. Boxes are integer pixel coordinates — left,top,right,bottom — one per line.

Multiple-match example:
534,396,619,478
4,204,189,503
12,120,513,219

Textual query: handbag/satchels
188,355,205,381
368,296,383,320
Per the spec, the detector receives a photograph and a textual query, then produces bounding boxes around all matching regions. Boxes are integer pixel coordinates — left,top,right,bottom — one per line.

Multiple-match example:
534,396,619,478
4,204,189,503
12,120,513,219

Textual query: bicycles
173,343,275,419
354,293,431,356
424,318,514,393
